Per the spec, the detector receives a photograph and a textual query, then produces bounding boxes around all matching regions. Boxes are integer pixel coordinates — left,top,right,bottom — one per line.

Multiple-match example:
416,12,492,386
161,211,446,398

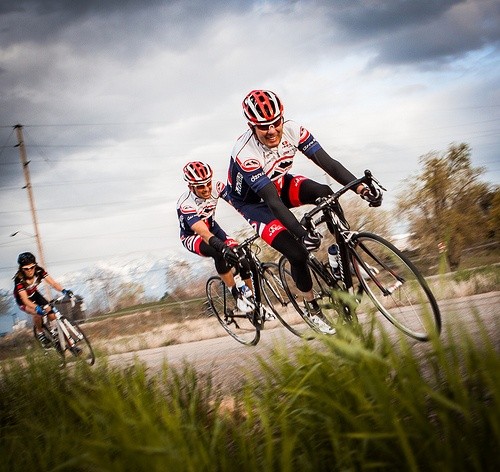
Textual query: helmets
18,252,35,265
241,90,283,125
183,161,213,183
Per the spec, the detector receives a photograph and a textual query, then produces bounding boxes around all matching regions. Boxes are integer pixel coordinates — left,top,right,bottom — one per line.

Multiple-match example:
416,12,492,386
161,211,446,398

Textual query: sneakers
65,342,82,357
303,294,337,335
348,260,379,281
37,332,52,348
234,293,255,312
260,306,276,320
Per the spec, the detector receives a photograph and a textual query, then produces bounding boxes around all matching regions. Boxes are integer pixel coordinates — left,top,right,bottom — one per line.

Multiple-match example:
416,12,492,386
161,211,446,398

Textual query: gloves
61,289,74,298
360,186,383,207
217,243,239,268
35,305,46,315
296,229,324,252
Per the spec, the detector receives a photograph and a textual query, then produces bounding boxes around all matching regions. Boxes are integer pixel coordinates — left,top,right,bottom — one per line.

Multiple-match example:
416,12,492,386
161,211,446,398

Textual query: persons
226,90,383,334
176,161,277,321
13,252,73,347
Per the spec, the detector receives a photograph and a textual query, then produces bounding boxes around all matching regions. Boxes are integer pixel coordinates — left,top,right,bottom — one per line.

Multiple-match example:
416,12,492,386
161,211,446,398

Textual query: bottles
327,243,338,268
232,272,252,299
54,328,59,343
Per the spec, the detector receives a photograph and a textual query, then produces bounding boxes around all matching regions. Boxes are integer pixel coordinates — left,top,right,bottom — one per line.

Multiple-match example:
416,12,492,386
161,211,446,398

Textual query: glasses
253,117,281,130
190,179,211,189
19,263,37,271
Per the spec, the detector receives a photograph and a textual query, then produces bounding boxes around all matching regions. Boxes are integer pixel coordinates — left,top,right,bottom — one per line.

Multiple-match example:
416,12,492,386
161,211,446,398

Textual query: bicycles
33,292,95,367
278,169,442,344
205,232,337,347
